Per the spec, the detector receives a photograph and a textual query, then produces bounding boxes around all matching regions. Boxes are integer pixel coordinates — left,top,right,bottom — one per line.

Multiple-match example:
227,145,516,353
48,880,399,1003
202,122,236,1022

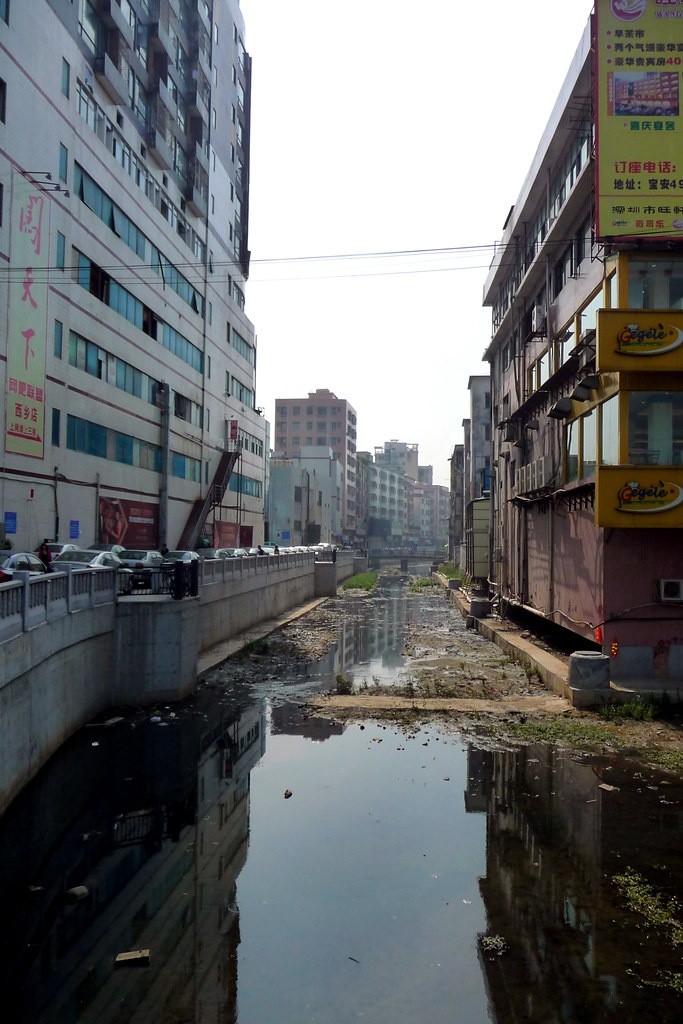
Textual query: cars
196,548,232,560
51,550,136,594
117,550,165,588
0,550,55,587
32,542,80,562
224,542,350,559
87,544,126,556
163,550,200,566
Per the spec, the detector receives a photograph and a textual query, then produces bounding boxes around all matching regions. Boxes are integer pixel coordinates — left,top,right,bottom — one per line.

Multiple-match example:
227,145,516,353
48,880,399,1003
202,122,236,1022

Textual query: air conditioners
516,456,553,496
532,305,545,332
493,548,501,561
660,579,683,601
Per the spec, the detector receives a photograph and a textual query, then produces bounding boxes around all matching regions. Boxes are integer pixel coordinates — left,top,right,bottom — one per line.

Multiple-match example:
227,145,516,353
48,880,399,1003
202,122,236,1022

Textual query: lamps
42,189,70,197
23,172,51,180
33,181,60,191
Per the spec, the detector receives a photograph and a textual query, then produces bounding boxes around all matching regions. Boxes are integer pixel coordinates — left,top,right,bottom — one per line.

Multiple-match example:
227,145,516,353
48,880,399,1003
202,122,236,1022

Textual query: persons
160,543,169,556
39,543,54,571
258,546,264,555
